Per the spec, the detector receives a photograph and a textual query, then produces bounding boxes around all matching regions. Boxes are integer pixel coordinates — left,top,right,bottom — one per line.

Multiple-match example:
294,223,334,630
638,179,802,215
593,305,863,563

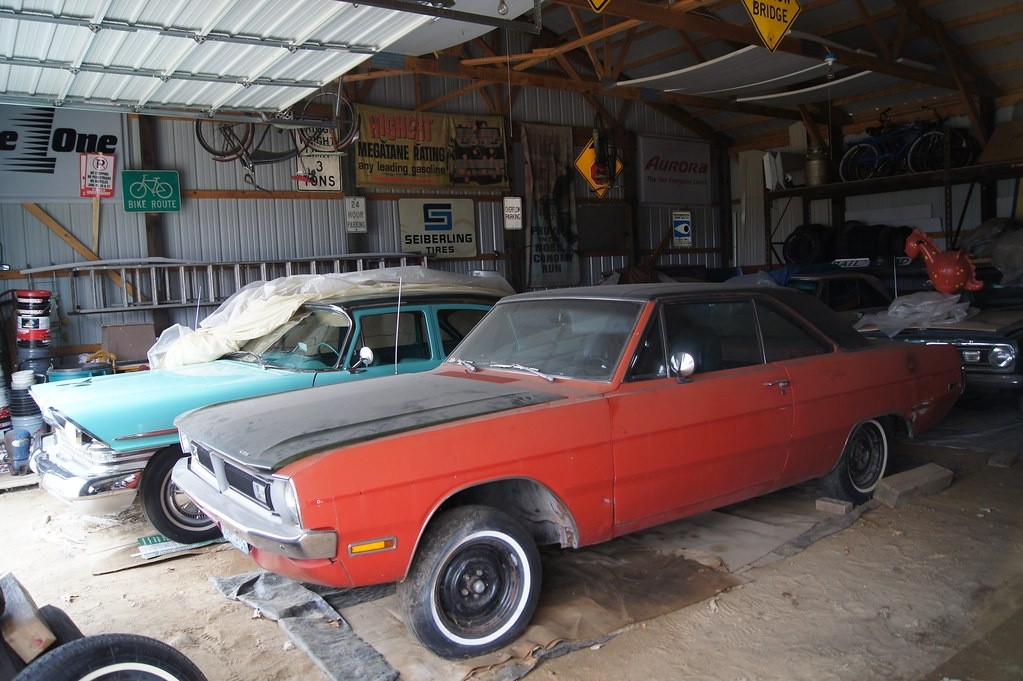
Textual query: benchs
719,335,826,363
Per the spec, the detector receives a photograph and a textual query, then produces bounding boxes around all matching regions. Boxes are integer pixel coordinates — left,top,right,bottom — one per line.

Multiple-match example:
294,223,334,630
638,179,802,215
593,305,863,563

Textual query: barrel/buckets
16,290,52,384
804,146,833,186
46,362,113,382
0,365,46,450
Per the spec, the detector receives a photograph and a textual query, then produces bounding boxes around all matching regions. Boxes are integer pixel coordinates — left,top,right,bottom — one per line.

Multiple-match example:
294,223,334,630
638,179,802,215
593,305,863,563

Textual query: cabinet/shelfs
762,152,1023,314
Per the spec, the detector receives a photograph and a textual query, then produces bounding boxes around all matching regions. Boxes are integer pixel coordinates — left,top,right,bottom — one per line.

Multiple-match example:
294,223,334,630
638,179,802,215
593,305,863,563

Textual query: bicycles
194,93,364,175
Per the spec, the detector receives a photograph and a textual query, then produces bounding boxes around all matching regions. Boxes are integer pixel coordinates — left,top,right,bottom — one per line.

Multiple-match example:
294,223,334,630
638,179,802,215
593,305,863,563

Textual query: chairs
670,327,724,371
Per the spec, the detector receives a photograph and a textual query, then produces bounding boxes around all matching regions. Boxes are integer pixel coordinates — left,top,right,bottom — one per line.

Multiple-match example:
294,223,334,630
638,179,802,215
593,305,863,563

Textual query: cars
599,255,1023,391
27,287,566,544
171,280,965,662
